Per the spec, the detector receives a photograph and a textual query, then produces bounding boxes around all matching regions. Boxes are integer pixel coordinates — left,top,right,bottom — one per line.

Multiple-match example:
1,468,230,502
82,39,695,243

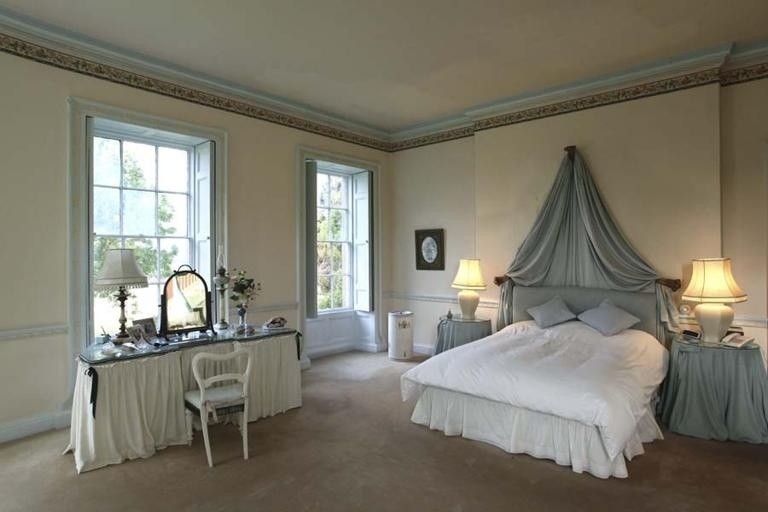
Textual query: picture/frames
415,229,444,270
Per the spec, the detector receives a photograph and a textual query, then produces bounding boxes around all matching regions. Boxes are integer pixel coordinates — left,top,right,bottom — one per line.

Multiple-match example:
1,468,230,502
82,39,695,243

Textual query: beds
413,286,660,471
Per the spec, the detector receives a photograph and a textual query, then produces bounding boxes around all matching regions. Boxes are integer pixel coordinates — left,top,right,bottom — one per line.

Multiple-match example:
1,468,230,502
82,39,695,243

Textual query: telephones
720,333,755,348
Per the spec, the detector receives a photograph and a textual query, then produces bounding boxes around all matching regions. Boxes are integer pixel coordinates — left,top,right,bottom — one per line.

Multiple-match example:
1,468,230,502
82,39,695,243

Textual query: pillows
525,292,642,336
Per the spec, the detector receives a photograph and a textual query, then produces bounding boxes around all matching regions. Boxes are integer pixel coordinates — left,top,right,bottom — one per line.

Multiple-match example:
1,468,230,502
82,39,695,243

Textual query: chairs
183,347,254,468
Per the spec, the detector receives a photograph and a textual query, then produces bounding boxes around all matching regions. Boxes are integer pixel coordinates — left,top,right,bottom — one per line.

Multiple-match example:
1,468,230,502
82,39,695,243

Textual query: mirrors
158,263,218,340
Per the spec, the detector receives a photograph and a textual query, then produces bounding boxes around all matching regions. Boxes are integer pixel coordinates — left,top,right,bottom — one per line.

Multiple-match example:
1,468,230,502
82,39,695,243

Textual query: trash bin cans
387,310,413,360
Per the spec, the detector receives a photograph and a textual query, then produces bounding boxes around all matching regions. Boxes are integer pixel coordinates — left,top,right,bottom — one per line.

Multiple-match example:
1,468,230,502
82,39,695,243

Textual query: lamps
451,258,487,321
95,248,148,344
681,257,749,347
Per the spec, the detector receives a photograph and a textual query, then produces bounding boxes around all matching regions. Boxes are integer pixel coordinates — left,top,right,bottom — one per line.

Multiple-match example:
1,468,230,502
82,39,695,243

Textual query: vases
238,309,247,330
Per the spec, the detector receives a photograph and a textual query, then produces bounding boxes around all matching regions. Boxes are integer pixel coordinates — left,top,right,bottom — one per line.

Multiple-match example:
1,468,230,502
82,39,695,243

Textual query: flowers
226,264,263,323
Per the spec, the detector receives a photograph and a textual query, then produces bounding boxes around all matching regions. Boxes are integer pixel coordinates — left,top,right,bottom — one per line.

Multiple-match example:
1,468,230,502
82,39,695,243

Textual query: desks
75,328,302,460
671,335,763,436
437,318,491,353
